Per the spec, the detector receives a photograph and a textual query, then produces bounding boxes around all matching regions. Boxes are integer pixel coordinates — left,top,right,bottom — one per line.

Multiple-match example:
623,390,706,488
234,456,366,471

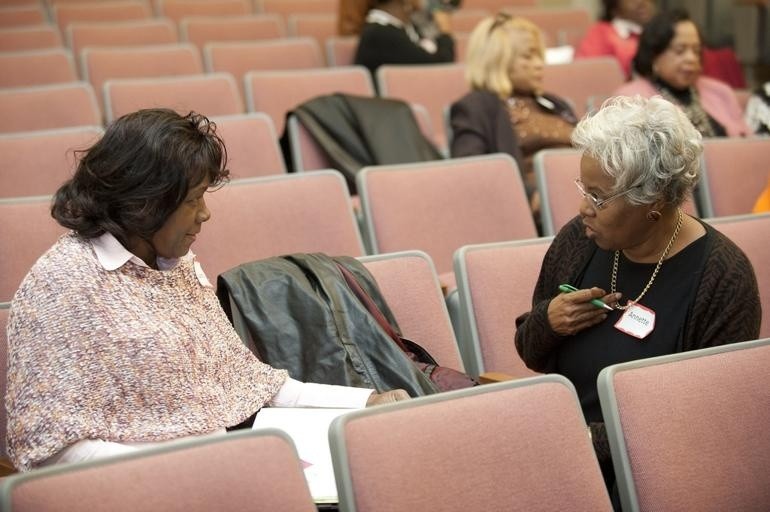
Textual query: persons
451,10,586,222
351,1,455,69
13,108,410,468
746,80,769,138
616,11,755,138
575,1,673,82
516,94,762,492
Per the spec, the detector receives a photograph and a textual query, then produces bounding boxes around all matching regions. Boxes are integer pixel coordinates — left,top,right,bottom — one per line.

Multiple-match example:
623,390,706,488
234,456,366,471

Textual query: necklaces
611,202,682,310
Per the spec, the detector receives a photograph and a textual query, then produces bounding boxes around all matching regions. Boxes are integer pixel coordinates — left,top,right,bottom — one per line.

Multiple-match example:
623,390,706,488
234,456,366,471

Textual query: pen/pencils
558,284,614,311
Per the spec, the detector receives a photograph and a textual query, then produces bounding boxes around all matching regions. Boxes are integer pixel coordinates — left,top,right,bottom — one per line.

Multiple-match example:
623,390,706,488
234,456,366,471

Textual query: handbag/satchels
393,339,485,392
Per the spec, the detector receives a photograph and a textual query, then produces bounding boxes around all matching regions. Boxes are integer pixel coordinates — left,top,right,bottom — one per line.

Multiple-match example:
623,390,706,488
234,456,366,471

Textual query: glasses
574,179,633,211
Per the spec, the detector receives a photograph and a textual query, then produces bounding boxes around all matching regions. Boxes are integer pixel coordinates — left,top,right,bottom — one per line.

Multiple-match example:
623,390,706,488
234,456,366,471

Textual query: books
249,404,367,512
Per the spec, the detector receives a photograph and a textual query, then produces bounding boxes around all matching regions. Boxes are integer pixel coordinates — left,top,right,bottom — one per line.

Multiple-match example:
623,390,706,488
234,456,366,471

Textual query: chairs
1,0,770,511
